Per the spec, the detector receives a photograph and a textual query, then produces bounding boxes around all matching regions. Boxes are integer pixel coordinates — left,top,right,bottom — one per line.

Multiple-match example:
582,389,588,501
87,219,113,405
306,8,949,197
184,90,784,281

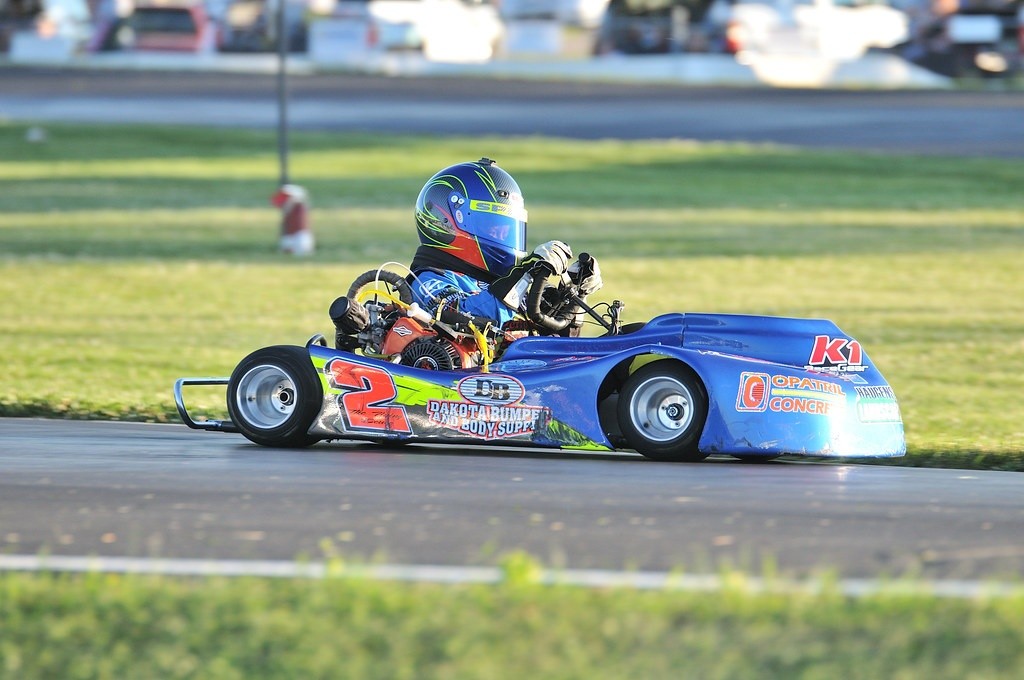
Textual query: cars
128,0,207,54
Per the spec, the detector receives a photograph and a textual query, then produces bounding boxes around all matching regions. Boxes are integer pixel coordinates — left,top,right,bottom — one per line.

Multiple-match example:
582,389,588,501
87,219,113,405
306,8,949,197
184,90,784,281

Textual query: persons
405,157,605,362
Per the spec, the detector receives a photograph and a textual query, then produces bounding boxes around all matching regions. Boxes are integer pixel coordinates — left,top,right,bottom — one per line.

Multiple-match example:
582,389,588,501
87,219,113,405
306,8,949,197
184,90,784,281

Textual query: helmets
414,157,529,277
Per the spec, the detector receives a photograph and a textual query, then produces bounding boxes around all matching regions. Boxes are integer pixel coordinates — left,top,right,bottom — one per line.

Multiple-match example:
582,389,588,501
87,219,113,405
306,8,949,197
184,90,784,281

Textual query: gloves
489,240,572,311
559,253,605,327
